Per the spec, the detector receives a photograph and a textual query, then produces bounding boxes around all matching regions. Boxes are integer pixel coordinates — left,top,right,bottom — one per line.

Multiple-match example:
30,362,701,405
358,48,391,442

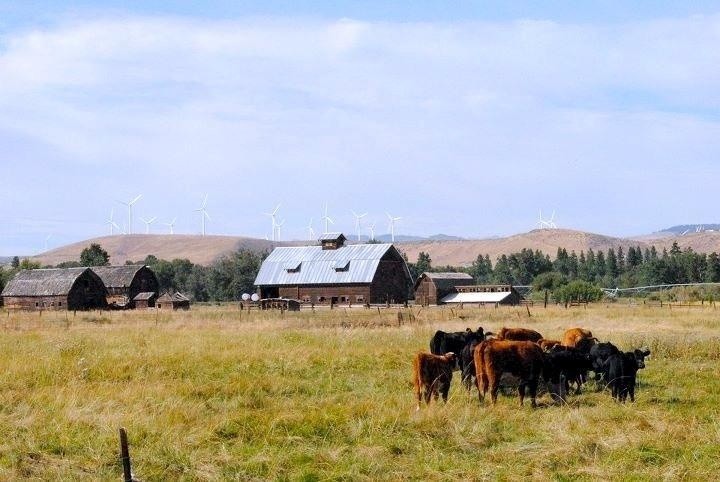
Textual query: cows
430,326,651,410
413,351,461,410
